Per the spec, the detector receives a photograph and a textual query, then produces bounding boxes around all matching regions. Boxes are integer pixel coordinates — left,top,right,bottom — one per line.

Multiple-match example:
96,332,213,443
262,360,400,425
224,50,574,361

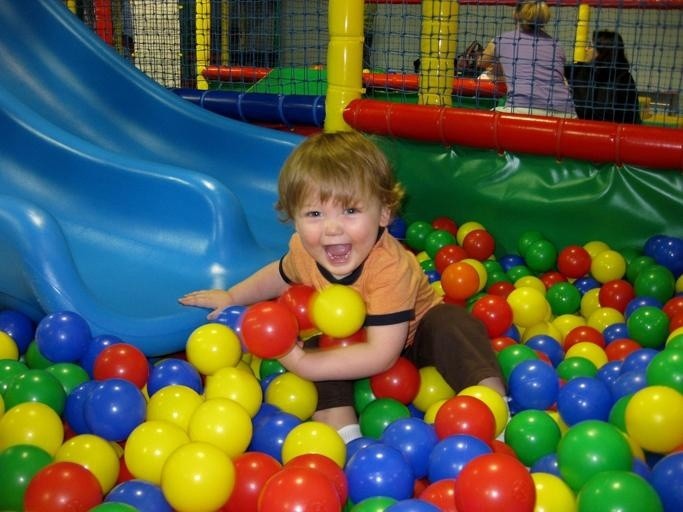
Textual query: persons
562,28,643,126
174,130,513,445
476,60,504,82
475,0,578,120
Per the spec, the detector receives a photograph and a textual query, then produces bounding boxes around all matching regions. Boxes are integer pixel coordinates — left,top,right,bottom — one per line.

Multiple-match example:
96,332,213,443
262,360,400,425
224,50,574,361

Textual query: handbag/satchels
454,40,485,77
